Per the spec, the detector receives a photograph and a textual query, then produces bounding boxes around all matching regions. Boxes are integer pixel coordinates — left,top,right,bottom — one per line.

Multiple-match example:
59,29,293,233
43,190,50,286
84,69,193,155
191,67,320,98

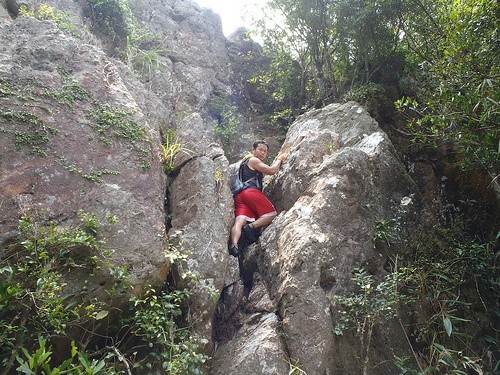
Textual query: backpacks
228,155,259,194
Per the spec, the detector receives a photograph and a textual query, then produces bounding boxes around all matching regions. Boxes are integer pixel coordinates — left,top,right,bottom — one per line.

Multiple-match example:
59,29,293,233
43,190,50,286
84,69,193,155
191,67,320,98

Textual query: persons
228,140,289,257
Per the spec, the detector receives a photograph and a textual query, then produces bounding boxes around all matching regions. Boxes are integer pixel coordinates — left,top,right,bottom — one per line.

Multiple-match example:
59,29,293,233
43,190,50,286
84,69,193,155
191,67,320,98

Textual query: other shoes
242,224,258,243
231,244,239,256
